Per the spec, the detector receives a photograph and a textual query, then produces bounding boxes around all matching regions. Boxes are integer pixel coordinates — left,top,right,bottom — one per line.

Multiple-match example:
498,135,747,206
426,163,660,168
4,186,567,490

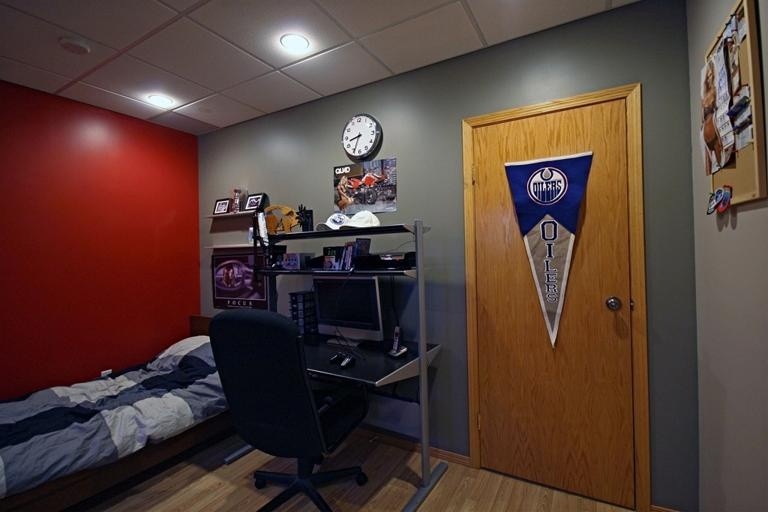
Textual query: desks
261,219,448,511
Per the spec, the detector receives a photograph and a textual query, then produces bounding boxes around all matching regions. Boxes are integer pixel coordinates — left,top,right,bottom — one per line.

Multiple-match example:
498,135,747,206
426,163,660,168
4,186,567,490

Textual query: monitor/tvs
311,273,416,347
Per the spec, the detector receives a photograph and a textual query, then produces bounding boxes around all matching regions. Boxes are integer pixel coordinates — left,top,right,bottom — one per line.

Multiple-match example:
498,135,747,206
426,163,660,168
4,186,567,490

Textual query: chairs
210,308,369,512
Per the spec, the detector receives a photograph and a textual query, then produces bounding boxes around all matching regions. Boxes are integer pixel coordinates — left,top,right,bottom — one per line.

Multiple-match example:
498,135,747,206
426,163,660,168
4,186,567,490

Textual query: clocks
342,113,382,159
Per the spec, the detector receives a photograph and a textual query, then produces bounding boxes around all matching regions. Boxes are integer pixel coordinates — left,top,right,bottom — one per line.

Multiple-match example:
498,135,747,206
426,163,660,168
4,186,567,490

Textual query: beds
0,315,239,512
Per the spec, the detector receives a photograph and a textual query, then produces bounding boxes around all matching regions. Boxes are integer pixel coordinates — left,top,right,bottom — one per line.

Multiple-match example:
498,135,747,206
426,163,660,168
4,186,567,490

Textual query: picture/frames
213,199,231,214
209,251,270,309
245,194,266,210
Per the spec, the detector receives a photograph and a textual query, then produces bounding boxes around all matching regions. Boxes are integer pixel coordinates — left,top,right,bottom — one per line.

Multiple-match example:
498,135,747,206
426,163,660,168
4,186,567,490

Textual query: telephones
387,323,408,358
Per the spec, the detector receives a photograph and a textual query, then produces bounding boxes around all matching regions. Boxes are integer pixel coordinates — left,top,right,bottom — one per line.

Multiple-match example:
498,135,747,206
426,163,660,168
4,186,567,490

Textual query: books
323,246,352,270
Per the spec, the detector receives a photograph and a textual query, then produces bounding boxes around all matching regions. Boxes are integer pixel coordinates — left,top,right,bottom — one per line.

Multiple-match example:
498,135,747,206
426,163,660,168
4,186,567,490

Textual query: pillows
151,335,215,369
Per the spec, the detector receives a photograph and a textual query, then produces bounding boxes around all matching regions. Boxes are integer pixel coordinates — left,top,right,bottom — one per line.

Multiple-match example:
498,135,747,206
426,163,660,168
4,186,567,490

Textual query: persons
361,168,383,187
700,61,723,177
337,176,354,214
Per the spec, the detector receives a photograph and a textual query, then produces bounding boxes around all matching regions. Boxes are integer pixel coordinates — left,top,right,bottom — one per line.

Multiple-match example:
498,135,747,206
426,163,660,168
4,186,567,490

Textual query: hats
315,212,350,230
339,210,380,230
